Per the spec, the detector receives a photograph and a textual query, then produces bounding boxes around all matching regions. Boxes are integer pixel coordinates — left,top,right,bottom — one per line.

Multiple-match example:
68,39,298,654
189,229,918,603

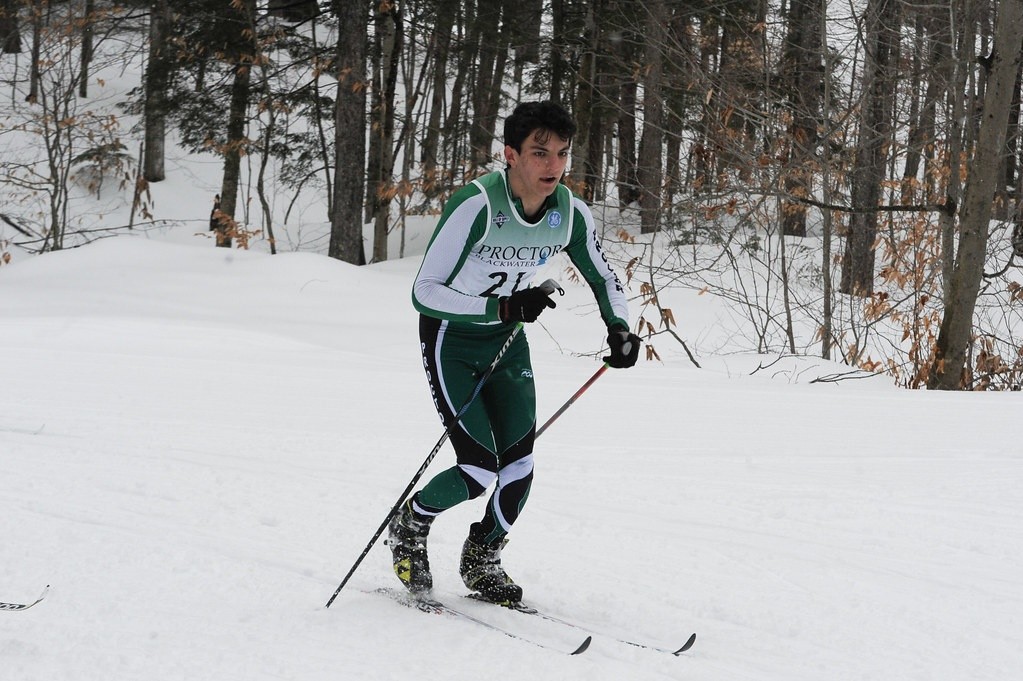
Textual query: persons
388,101,643,601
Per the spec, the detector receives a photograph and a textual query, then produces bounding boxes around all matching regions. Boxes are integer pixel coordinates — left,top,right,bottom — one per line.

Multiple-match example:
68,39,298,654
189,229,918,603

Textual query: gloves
498,286,556,323
602,323,643,369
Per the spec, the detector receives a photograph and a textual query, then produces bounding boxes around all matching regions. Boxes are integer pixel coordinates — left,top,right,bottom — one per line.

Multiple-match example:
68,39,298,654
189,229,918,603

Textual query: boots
388,494,435,596
459,522,523,607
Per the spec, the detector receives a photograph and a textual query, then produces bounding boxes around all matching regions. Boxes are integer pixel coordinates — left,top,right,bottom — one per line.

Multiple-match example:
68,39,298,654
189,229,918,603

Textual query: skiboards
374,583,698,657
